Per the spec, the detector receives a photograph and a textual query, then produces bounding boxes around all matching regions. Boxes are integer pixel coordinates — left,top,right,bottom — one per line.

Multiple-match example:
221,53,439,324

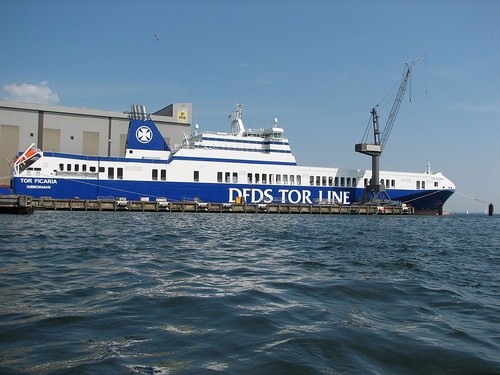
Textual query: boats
0,103,457,216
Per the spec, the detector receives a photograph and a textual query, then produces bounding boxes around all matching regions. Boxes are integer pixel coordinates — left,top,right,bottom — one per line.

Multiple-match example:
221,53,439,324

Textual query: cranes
354,55,426,206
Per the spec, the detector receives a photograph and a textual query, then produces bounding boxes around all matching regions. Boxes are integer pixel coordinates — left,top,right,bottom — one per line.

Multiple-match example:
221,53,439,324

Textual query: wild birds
151,34,158,42
